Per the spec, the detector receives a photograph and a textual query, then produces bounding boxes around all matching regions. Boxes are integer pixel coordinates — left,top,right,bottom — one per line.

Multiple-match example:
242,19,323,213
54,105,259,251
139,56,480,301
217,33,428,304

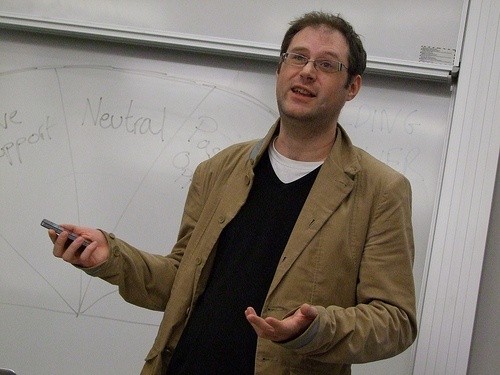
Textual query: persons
47,9,418,375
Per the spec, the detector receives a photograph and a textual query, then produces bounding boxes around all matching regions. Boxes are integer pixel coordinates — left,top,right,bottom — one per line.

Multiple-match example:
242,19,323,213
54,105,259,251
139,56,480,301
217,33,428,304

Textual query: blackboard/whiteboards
0,0,472,375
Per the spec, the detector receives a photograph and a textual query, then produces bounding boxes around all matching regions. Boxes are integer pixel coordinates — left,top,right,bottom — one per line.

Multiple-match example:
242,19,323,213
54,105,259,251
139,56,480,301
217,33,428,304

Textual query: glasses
282,52,348,73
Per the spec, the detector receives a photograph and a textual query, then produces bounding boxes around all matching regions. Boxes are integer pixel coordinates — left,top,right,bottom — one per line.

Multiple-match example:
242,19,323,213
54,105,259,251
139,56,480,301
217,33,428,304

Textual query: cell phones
40,219,93,247
